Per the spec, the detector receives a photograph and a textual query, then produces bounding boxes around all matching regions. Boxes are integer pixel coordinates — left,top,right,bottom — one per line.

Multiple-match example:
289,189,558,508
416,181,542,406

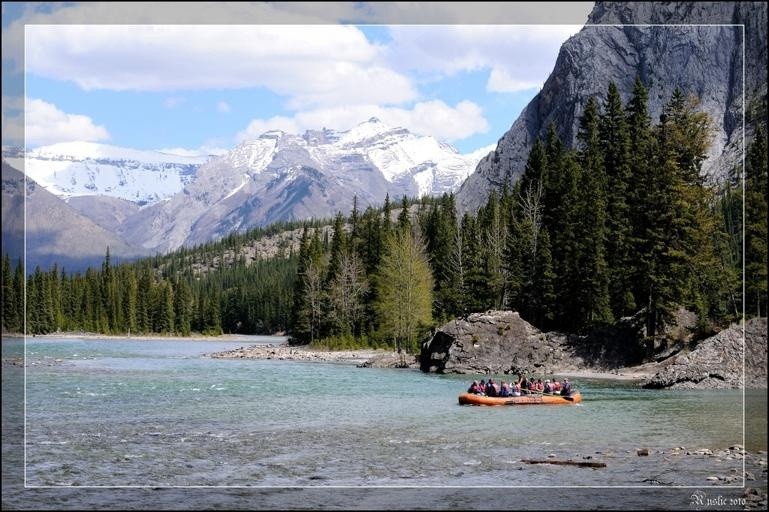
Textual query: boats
458,389,581,406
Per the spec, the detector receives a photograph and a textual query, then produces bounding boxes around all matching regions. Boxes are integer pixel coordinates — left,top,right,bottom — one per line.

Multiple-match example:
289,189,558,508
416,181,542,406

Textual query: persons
469,372,571,395
